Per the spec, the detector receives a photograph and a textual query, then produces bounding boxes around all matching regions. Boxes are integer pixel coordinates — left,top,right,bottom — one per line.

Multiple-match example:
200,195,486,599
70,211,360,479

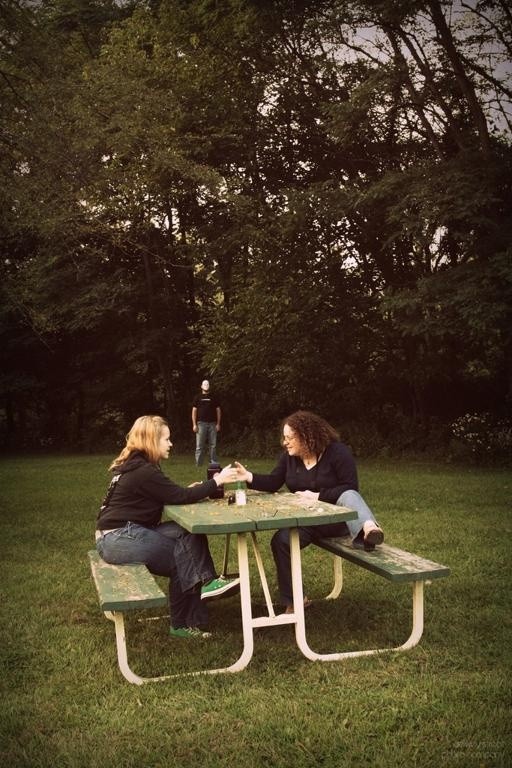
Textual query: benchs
307,532,452,662
87,546,170,687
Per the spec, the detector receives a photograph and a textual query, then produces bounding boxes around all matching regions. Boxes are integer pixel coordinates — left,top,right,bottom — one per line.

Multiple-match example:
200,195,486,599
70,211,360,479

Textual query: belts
94,528,118,544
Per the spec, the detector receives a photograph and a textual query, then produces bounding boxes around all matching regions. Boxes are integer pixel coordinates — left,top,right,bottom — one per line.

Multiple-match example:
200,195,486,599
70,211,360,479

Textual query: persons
192,380,223,465
95,413,241,639
234,410,384,617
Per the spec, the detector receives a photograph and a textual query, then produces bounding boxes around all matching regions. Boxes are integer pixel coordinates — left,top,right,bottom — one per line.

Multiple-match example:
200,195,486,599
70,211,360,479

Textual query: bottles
235,481,247,505
206,463,224,499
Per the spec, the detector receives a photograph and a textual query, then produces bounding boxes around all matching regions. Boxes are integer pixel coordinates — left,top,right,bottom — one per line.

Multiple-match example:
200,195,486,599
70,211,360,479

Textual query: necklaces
305,454,316,466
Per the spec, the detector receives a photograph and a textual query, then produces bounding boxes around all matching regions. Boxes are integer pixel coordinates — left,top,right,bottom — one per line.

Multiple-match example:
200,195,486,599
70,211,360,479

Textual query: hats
200,380,210,391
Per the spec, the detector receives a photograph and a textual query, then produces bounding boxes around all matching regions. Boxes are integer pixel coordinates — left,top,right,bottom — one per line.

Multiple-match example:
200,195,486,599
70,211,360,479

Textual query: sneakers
199,574,240,602
362,526,385,554
168,624,212,639
283,594,314,617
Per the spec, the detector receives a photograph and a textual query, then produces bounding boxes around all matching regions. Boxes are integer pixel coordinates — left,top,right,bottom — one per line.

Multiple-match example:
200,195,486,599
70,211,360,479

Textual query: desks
159,487,360,682
